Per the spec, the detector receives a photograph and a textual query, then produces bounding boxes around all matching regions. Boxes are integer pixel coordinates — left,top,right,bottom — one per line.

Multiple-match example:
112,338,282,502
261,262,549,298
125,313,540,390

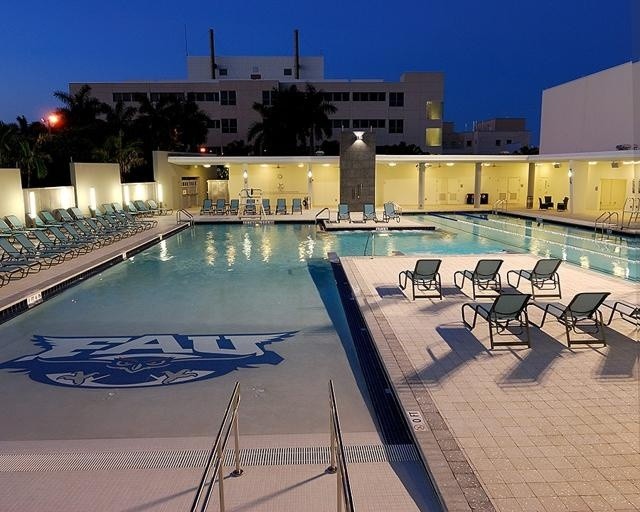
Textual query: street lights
39,115,56,135
